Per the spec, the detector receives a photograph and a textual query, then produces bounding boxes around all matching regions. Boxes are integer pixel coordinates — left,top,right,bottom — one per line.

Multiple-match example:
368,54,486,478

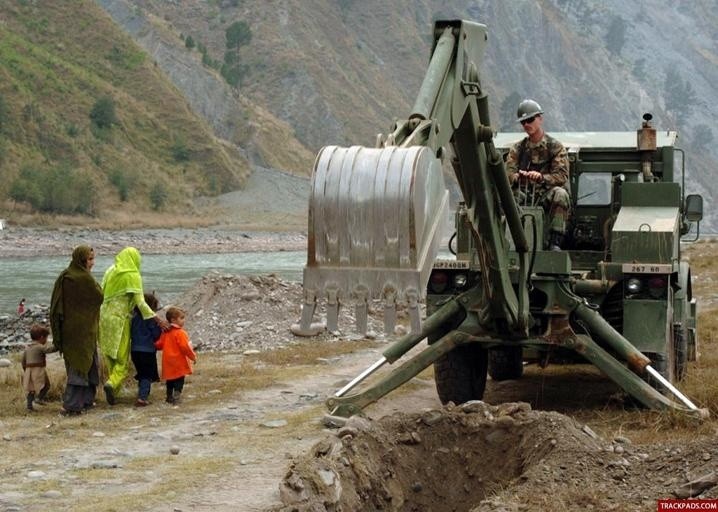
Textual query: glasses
520,114,540,125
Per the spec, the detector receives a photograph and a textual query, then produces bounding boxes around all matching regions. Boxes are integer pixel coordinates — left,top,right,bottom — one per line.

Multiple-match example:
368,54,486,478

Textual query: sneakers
134,395,183,407
59,398,101,417
27,399,47,413
103,383,116,406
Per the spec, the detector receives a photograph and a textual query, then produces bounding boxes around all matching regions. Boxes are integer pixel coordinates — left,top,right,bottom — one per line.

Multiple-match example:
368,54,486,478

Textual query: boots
548,227,564,252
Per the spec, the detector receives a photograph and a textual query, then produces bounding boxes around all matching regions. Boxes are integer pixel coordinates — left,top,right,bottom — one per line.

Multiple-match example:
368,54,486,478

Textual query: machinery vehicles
298,16,716,427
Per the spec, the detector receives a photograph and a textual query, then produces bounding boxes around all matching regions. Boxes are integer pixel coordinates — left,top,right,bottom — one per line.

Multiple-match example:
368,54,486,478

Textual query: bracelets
157,319,163,326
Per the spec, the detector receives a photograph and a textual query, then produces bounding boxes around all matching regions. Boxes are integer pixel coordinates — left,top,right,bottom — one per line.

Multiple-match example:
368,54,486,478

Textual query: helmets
516,99,544,122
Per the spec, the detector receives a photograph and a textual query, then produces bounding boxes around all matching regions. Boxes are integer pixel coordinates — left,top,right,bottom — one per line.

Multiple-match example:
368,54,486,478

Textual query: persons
504,99,571,252
152,307,197,405
20,325,59,414
128,293,161,406
49,245,104,418
97,247,172,404
15,297,27,315
